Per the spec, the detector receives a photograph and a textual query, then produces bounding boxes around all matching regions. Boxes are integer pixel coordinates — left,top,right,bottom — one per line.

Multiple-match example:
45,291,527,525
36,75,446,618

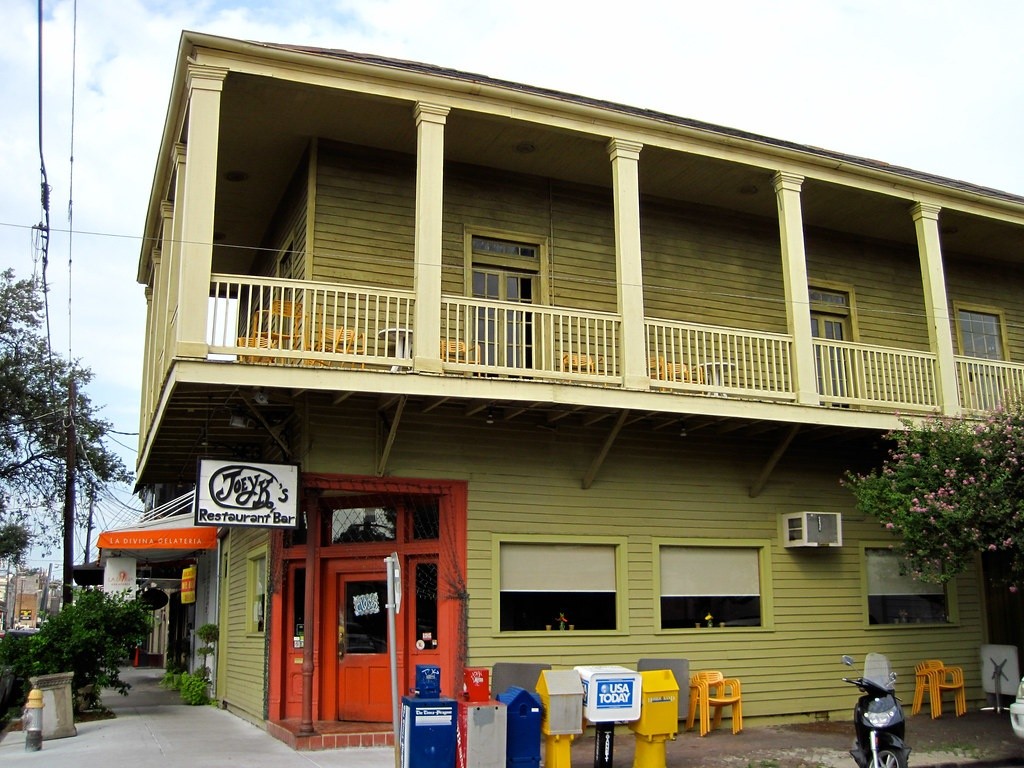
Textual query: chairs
237,300,367,369
911,660,967,719
645,357,690,394
440,340,481,378
560,354,607,386
686,672,743,737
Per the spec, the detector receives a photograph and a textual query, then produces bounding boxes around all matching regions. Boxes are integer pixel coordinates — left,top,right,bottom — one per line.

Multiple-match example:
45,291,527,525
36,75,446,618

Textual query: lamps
229,415,256,430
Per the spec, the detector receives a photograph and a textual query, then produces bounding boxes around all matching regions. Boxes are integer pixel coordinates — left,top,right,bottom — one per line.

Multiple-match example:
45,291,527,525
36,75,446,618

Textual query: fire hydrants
18,685,46,752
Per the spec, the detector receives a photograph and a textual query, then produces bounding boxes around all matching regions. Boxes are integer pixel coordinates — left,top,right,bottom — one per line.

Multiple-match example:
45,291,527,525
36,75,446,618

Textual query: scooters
840,652,912,768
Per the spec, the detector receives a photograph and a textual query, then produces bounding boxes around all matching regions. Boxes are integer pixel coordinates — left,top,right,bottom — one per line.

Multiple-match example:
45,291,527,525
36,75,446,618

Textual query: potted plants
180,671,209,704
158,660,191,690
12,585,144,710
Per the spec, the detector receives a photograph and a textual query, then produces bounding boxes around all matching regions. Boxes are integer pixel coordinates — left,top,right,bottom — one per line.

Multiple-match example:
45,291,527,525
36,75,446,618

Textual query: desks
696,363,736,397
492,662,551,704
635,660,690,720
979,645,1020,714
378,327,415,371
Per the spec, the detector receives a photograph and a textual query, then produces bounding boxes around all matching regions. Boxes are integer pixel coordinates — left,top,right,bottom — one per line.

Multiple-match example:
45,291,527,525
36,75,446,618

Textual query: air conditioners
782,510,845,548
